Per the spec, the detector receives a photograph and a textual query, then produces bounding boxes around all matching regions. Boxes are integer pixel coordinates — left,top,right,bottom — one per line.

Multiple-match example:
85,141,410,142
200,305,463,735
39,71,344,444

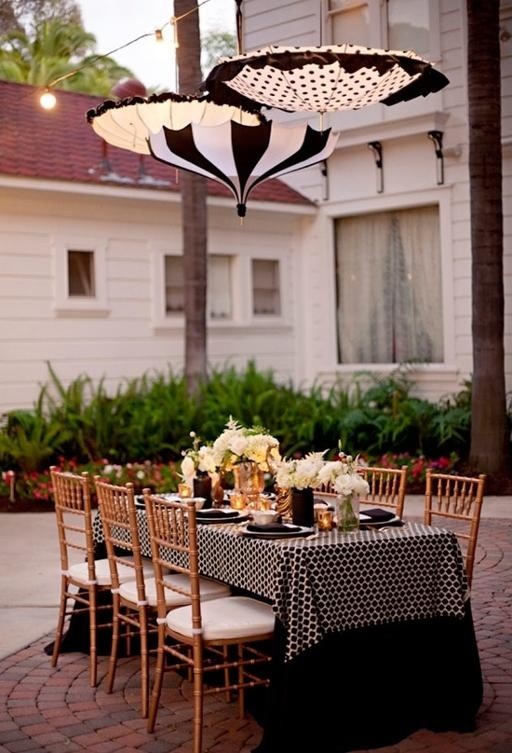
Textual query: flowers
179,409,376,530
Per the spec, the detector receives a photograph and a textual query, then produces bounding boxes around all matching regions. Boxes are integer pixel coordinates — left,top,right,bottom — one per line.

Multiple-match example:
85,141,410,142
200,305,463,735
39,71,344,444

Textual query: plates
135,495,248,520
239,521,314,538
314,499,399,526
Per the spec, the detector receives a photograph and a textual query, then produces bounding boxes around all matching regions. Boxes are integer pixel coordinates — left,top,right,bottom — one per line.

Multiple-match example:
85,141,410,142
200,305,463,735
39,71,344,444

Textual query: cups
252,509,276,527
317,509,332,534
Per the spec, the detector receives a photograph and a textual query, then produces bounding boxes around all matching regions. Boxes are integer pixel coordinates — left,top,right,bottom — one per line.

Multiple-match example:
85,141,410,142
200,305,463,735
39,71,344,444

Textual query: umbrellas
86,14,265,182
202,0,448,134
138,105,342,225
